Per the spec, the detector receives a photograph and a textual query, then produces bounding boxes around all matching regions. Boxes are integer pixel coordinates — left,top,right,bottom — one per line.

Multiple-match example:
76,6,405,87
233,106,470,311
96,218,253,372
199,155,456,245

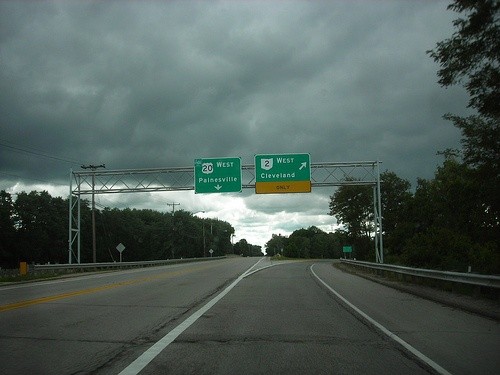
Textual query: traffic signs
194,157,242,193
254,153,311,194
